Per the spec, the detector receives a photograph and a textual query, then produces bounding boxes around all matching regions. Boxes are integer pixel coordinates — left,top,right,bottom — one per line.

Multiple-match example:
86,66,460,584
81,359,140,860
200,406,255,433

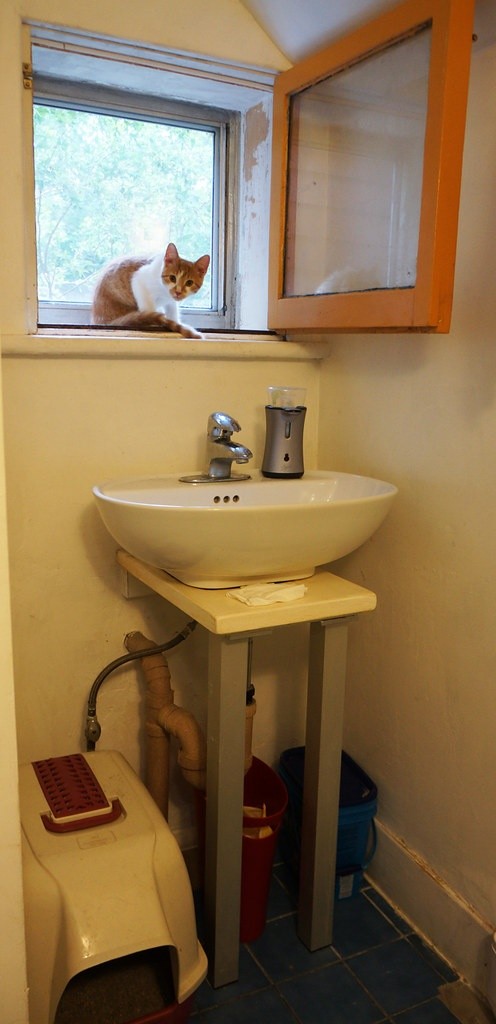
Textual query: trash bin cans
273,741,377,902
197,754,289,942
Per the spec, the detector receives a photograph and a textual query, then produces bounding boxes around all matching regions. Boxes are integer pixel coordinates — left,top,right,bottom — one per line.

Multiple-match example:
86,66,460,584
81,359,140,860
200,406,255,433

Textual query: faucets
206,412,253,477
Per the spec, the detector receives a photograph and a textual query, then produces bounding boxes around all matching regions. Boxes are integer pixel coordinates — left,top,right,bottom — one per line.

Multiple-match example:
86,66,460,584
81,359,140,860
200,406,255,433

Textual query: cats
92,243,211,339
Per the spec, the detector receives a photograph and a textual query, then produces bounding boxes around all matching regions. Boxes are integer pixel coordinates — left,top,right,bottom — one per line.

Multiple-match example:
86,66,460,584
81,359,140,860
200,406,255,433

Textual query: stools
19,749,209,1024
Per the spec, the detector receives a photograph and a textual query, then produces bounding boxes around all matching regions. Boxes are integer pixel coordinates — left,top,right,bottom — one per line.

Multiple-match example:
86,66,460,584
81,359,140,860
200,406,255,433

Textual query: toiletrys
260,386,307,478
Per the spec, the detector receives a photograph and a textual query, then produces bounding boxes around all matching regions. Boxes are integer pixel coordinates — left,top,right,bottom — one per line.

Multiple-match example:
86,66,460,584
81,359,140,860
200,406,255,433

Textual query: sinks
91,467,400,589
